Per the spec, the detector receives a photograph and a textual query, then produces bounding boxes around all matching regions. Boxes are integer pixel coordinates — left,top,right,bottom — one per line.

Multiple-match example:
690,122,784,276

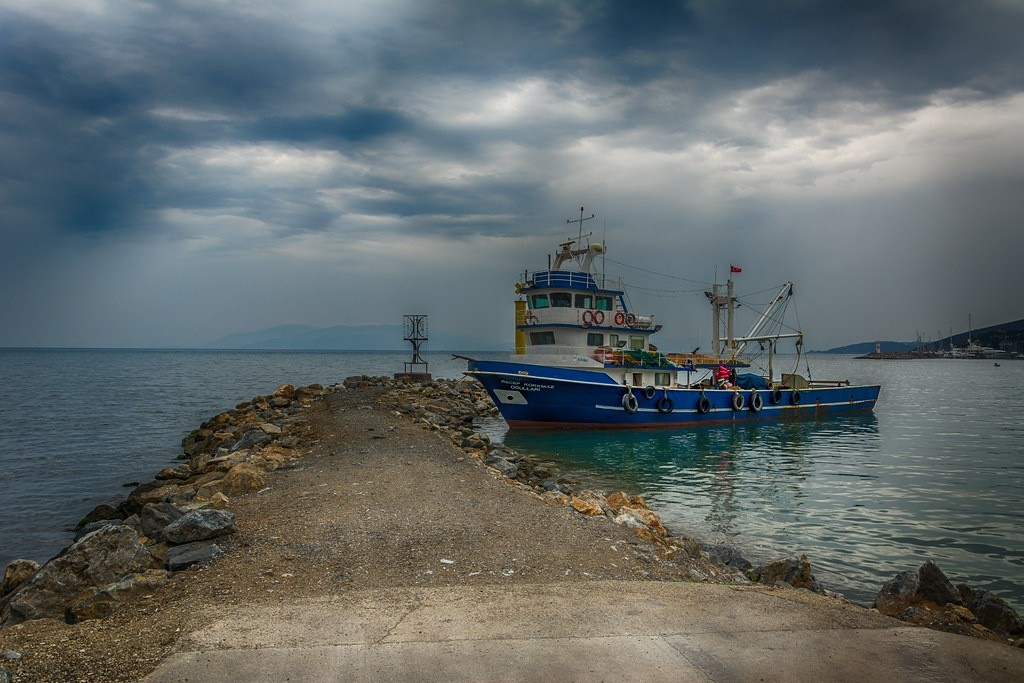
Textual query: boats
451,207,881,430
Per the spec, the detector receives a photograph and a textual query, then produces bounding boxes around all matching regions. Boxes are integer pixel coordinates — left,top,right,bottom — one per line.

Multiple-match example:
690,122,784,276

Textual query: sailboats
911,313,1018,359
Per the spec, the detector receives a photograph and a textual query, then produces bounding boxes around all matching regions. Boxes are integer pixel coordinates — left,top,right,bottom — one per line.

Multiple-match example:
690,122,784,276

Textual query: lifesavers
614,312,624,326
593,310,605,324
583,309,594,325
749,394,763,413
771,390,783,405
626,312,635,327
658,397,674,414
697,397,711,414
622,394,639,415
645,386,656,400
789,391,802,405
732,393,745,412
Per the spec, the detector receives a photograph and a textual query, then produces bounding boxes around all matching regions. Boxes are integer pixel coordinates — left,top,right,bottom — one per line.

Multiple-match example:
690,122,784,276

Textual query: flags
731,266,742,273
